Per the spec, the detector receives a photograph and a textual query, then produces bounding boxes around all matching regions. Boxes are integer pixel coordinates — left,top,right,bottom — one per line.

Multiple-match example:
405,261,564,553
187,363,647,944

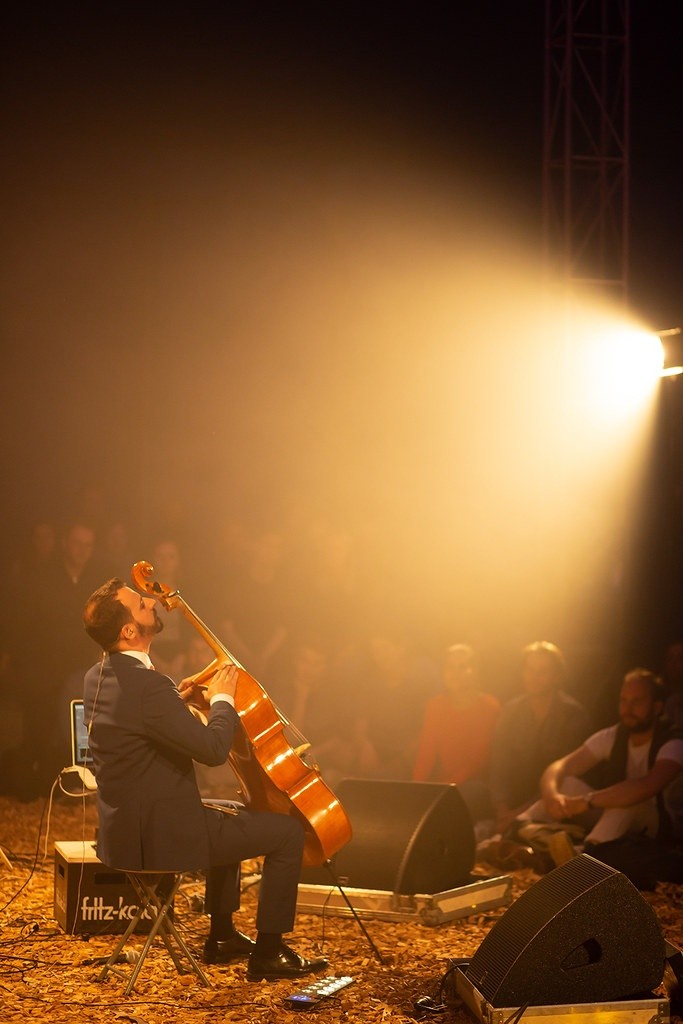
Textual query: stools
100,857,214,997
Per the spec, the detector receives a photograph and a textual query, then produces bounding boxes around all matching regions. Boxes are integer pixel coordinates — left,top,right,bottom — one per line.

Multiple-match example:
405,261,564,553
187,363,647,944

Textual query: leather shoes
246,946,329,982
202,932,256,963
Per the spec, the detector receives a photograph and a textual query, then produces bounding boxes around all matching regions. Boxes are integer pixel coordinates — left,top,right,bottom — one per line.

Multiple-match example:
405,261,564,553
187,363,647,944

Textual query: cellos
128,558,387,969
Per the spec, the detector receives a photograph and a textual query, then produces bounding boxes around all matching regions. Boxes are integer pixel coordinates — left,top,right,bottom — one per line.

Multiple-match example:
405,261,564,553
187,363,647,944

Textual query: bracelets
584,792,593,809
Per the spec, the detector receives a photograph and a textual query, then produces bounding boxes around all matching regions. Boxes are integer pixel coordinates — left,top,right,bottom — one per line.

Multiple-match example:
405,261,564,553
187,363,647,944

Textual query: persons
411,645,505,824
1,523,408,782
508,666,683,888
659,643,681,738
82,578,329,983
474,642,592,842
